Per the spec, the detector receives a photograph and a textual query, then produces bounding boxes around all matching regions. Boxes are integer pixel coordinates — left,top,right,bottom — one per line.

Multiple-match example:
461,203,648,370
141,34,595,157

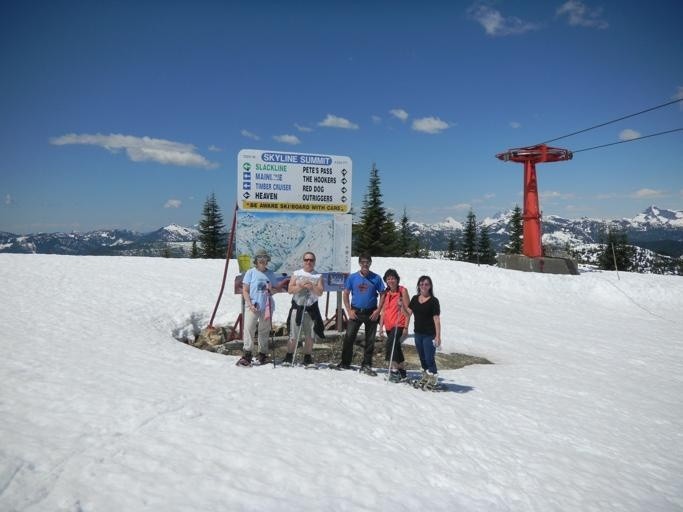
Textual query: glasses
303,258,313,262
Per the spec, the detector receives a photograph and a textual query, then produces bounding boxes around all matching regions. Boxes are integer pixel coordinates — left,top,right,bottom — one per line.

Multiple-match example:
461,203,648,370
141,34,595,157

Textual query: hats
254,248,270,262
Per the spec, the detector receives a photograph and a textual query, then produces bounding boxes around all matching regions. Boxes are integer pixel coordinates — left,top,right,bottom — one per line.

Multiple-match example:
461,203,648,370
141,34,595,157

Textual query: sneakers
336,361,351,369
359,362,377,377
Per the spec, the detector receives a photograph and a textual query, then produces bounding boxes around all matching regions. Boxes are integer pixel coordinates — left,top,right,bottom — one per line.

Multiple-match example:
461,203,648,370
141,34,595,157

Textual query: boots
281,352,293,364
385,367,440,390
254,351,267,364
303,355,313,365
239,352,252,365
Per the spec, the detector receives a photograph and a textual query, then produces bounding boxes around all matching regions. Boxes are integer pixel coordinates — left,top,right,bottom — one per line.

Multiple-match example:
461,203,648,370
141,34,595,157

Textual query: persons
336,251,385,377
237,247,291,366
396,275,442,390
281,251,325,366
378,268,411,383
